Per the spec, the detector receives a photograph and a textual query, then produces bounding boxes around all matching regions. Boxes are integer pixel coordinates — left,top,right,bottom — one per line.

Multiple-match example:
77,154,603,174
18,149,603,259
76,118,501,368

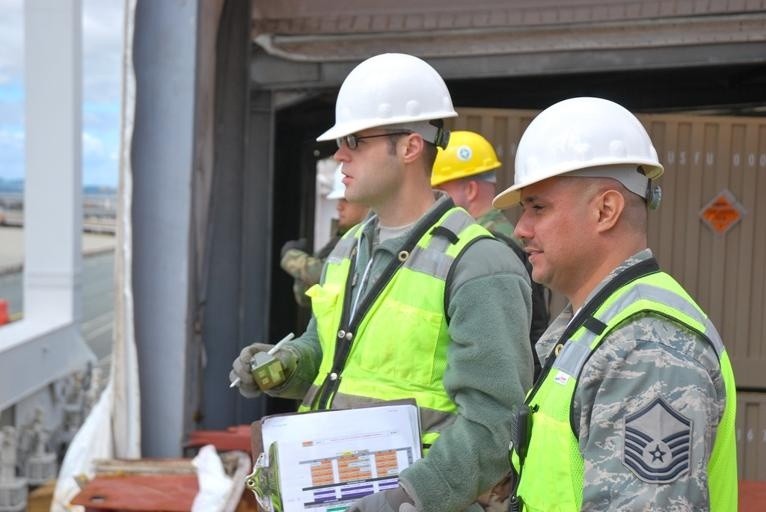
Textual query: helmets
428,130,503,188
326,161,350,200
314,52,459,142
491,94,664,211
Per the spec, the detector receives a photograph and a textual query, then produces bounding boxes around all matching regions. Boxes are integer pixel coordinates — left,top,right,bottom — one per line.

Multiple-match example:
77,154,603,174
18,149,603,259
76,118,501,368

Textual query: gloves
278,235,308,260
226,343,301,399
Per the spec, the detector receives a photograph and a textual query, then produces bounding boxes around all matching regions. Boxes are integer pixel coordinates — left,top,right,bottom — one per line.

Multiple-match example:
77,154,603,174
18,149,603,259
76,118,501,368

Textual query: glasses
332,132,413,150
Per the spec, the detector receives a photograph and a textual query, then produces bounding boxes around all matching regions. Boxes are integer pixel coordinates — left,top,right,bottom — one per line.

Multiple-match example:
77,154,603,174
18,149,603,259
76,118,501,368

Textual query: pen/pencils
229,332,295,389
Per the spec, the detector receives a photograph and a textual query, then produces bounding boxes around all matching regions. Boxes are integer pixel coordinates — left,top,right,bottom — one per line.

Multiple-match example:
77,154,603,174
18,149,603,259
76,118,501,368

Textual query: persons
277,160,374,313
426,129,550,380
227,51,537,512
493,95,742,512
